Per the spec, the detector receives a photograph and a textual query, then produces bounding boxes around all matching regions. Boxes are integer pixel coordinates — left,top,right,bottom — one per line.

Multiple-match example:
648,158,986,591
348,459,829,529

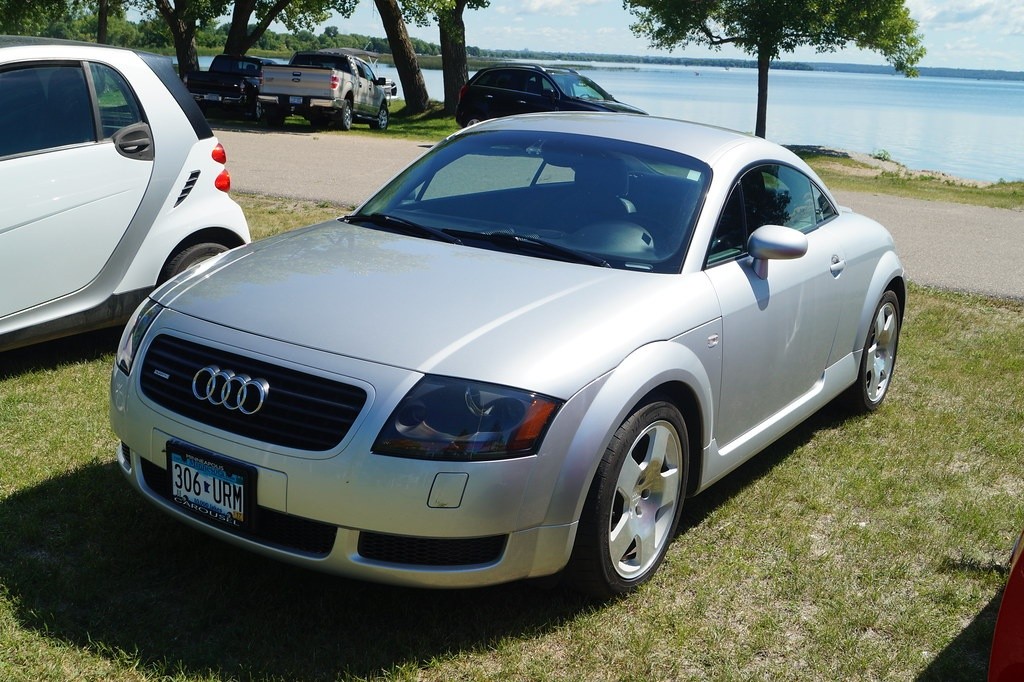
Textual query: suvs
455,63,650,128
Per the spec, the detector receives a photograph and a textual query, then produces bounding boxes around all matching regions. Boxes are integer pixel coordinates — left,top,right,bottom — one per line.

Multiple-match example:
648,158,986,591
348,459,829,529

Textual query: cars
0,36,251,354
110,111,908,593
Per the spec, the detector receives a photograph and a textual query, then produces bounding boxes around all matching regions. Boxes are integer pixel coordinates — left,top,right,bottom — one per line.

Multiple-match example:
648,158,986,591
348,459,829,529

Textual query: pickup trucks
184,53,278,124
256,52,389,131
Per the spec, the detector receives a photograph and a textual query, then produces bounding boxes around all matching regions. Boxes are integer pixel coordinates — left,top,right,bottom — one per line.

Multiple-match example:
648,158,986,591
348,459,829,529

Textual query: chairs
558,80,572,97
246,65,256,73
558,158,635,233
528,81,541,94
665,172,768,255
29,68,93,150
